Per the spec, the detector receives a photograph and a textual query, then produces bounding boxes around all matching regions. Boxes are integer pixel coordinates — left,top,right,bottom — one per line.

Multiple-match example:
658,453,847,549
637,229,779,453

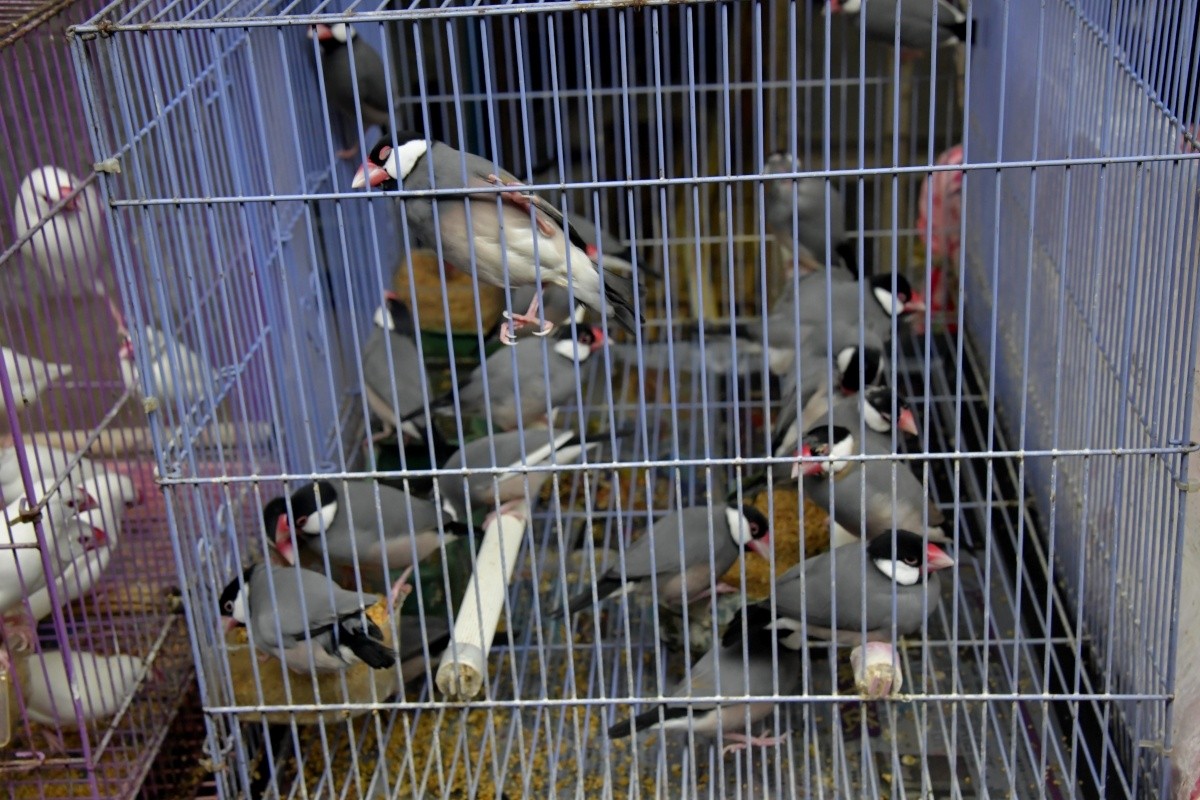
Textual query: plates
204,626,397,725
392,248,504,334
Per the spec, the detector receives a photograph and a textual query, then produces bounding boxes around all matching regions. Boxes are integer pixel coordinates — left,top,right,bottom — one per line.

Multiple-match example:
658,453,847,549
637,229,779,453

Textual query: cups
0,670,13,749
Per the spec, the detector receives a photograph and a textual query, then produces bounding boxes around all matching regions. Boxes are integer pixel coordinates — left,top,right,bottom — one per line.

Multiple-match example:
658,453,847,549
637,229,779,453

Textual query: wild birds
261,480,484,575
819,0,976,49
352,130,664,347
305,21,401,160
609,635,802,753
220,562,398,678
0,346,154,680
17,648,165,759
14,166,133,335
432,266,647,530
728,345,978,559
547,504,769,632
362,289,449,457
724,426,954,651
120,325,225,401
684,149,926,375
